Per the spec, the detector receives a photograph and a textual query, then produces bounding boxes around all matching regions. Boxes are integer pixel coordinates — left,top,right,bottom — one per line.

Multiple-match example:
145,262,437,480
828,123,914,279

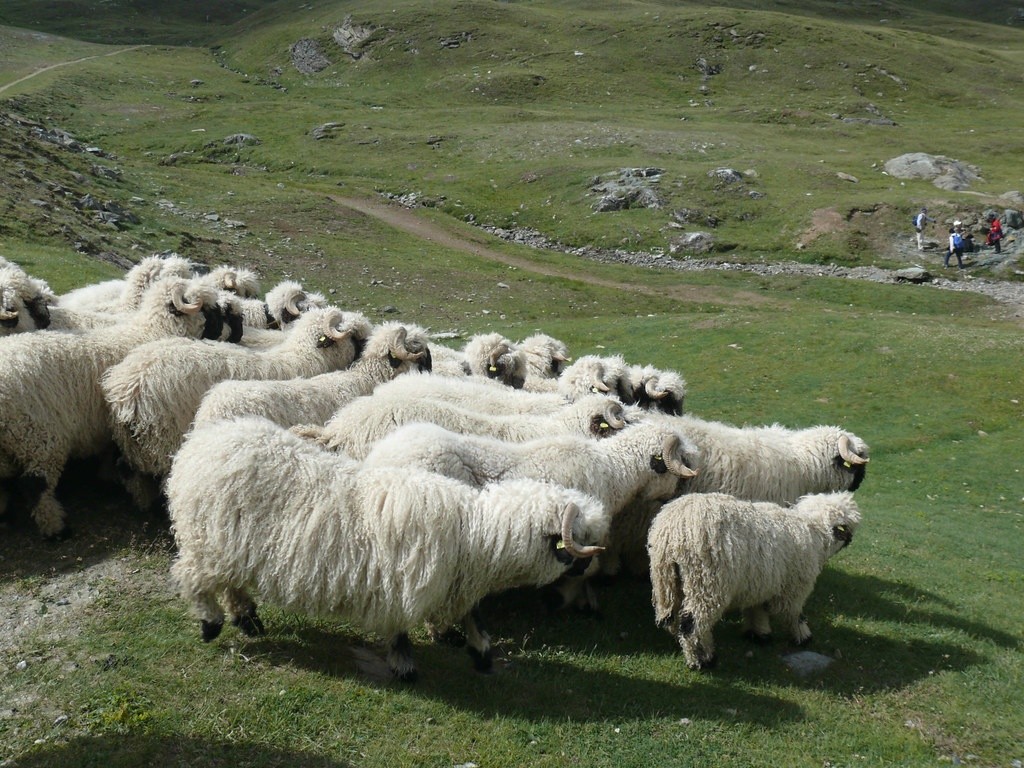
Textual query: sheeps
318,355,871,672
0,255,370,534
164,323,608,688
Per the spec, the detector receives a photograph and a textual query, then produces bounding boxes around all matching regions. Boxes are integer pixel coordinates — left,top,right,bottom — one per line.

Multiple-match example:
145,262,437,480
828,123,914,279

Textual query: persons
943,213,1003,269
916,208,937,252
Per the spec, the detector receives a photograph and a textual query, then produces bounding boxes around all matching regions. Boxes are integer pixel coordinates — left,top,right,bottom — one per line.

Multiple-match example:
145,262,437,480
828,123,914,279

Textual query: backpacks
985,231,993,245
952,233,964,251
912,216,918,226
